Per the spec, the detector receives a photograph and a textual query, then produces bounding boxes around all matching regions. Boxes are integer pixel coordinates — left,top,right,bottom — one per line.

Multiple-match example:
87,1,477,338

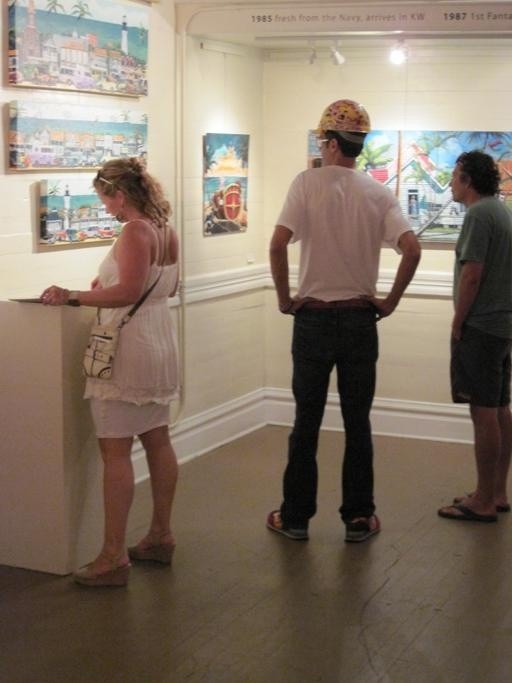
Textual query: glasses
95,171,113,185
316,137,328,147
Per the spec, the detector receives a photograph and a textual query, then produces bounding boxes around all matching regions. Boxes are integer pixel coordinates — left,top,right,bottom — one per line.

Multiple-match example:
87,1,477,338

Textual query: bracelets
282,300,294,313
68,290,81,308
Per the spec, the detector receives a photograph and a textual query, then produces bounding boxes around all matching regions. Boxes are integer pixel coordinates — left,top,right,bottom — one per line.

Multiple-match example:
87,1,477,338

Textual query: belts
305,301,366,308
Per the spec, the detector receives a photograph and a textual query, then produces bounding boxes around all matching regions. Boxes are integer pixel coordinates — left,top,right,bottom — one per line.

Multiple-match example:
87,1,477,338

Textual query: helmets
310,99,370,134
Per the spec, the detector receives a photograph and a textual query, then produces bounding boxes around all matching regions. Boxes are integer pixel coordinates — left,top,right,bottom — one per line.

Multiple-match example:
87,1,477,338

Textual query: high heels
73,551,131,586
127,530,175,565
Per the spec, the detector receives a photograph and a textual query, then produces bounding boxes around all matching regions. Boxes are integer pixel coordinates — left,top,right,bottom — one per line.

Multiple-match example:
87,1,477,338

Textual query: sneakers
345,515,381,541
267,510,308,540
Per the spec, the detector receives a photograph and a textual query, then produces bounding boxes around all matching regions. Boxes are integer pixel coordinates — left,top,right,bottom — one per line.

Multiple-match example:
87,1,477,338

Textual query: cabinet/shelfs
1,301,105,575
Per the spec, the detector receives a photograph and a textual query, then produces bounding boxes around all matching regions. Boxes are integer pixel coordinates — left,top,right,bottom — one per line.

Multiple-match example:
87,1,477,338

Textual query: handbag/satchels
81,326,119,379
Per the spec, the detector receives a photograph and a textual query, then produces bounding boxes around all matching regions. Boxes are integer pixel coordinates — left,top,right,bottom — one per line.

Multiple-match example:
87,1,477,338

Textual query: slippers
438,503,496,522
454,493,509,512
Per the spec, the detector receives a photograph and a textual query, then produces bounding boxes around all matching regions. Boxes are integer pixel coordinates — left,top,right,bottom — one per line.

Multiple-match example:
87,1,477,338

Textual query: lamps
329,47,350,66
390,42,407,66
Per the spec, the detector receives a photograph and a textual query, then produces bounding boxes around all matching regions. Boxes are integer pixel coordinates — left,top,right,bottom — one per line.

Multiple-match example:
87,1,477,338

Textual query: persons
437,149,512,521
267,100,421,542
40,158,178,587
